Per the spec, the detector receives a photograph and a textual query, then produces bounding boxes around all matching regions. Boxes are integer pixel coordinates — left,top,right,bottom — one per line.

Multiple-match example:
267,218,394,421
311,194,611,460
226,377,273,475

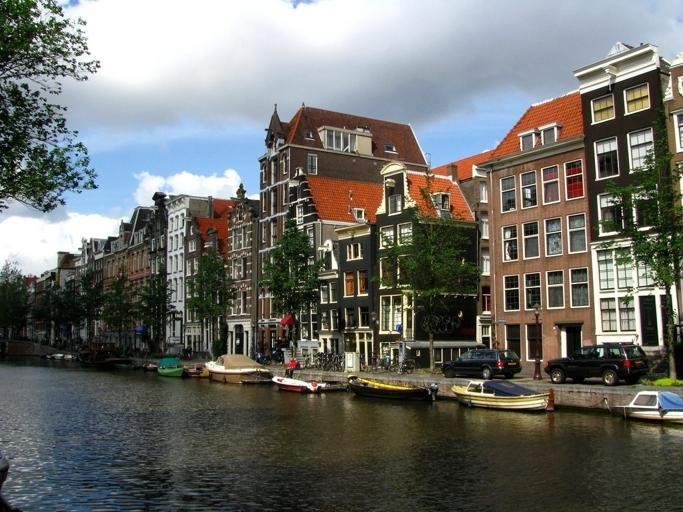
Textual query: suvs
543,341,649,385
440,348,521,379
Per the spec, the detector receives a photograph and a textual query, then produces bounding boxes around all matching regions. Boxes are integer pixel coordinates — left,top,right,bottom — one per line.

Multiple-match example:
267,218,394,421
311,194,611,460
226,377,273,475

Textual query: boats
203,354,271,384
346,374,438,401
272,375,329,392
608,390,683,424
449,381,554,409
157,365,183,377
45,342,132,366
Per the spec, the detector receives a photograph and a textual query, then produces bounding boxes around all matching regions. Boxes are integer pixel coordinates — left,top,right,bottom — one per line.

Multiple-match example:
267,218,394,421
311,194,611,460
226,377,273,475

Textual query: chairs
608,352,616,359
586,352,599,360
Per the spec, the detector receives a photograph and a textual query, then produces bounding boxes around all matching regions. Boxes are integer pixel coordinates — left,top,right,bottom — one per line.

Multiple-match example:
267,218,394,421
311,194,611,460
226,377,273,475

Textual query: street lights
371,311,377,357
532,302,541,358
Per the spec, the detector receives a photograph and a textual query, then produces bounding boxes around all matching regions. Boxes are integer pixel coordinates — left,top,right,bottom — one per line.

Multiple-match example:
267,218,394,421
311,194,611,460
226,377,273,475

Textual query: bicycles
304,349,345,372
357,352,415,375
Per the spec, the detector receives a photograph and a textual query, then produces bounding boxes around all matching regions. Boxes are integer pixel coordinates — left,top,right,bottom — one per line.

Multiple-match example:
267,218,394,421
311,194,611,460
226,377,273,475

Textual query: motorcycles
254,345,283,365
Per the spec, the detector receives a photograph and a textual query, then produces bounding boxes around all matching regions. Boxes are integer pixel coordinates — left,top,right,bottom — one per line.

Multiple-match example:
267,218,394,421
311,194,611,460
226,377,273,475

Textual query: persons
293,358,298,367
284,358,296,378
